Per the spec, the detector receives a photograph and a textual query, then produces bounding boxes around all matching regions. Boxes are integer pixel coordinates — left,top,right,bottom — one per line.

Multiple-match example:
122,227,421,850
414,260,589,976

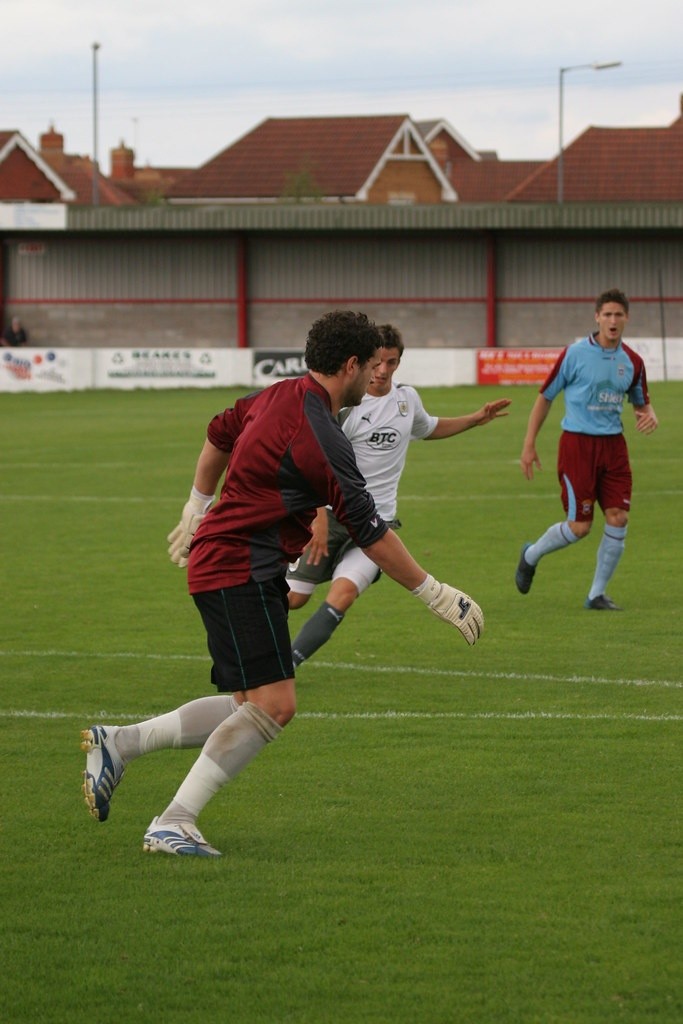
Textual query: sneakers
80,724,126,823
582,594,624,611
142,815,222,856
515,543,537,595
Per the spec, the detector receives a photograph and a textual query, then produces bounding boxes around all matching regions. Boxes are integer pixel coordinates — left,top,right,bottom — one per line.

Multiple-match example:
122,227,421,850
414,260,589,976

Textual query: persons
80,309,484,860
515,289,658,611
0,315,28,348
279,321,513,669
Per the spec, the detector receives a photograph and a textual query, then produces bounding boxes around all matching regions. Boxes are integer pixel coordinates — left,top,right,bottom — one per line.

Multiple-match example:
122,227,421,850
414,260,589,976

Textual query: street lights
90,43,100,207
557,60,621,203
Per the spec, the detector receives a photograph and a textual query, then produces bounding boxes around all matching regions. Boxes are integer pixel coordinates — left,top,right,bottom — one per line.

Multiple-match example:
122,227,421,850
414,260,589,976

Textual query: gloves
413,574,484,646
165,491,212,568
